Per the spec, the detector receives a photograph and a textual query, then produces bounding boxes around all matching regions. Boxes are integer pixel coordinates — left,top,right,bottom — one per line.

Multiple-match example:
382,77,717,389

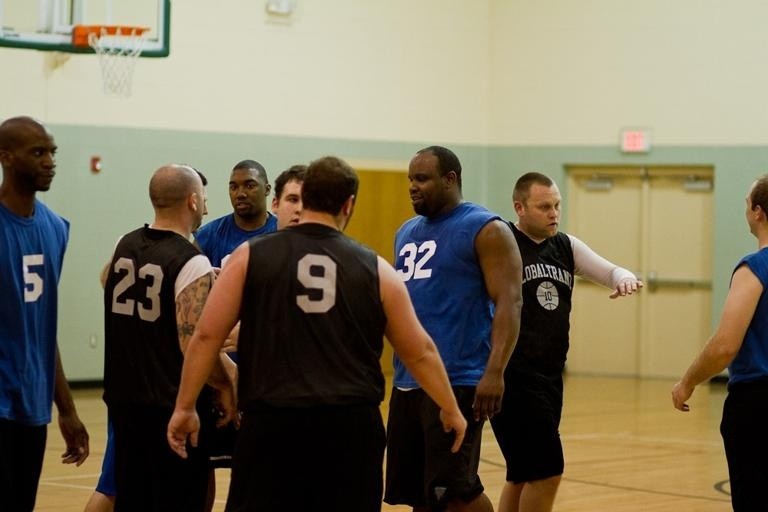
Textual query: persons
0,117,90,512
672,176,768,512
